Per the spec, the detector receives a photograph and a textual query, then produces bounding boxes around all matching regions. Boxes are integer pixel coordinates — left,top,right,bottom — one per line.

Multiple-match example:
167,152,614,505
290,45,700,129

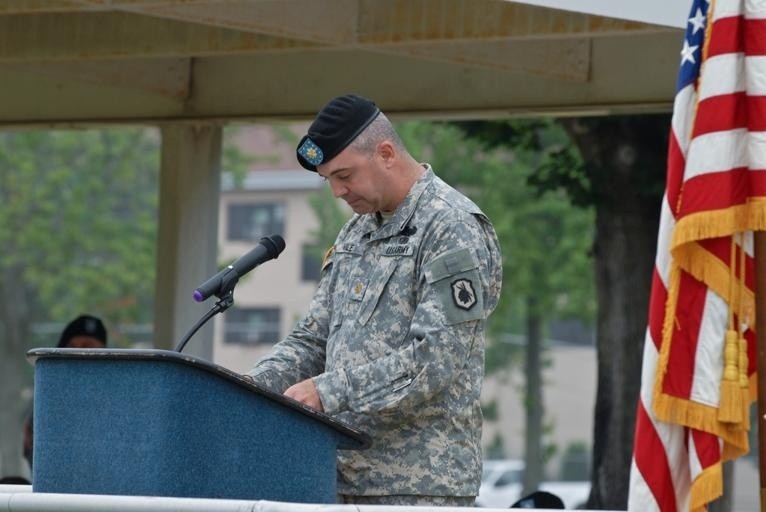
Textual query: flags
627,1,766,512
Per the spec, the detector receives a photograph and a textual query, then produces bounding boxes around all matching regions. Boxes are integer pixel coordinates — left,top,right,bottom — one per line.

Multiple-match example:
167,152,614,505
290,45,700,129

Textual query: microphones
193,235,286,302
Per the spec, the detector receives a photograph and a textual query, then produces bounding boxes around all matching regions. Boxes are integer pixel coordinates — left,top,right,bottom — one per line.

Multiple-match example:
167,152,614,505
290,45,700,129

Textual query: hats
57,315,106,348
296,94,380,171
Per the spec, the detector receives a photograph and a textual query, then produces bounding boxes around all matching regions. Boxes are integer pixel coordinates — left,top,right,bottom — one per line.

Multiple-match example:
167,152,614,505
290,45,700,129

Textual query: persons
233,92,502,507
23,315,106,472
